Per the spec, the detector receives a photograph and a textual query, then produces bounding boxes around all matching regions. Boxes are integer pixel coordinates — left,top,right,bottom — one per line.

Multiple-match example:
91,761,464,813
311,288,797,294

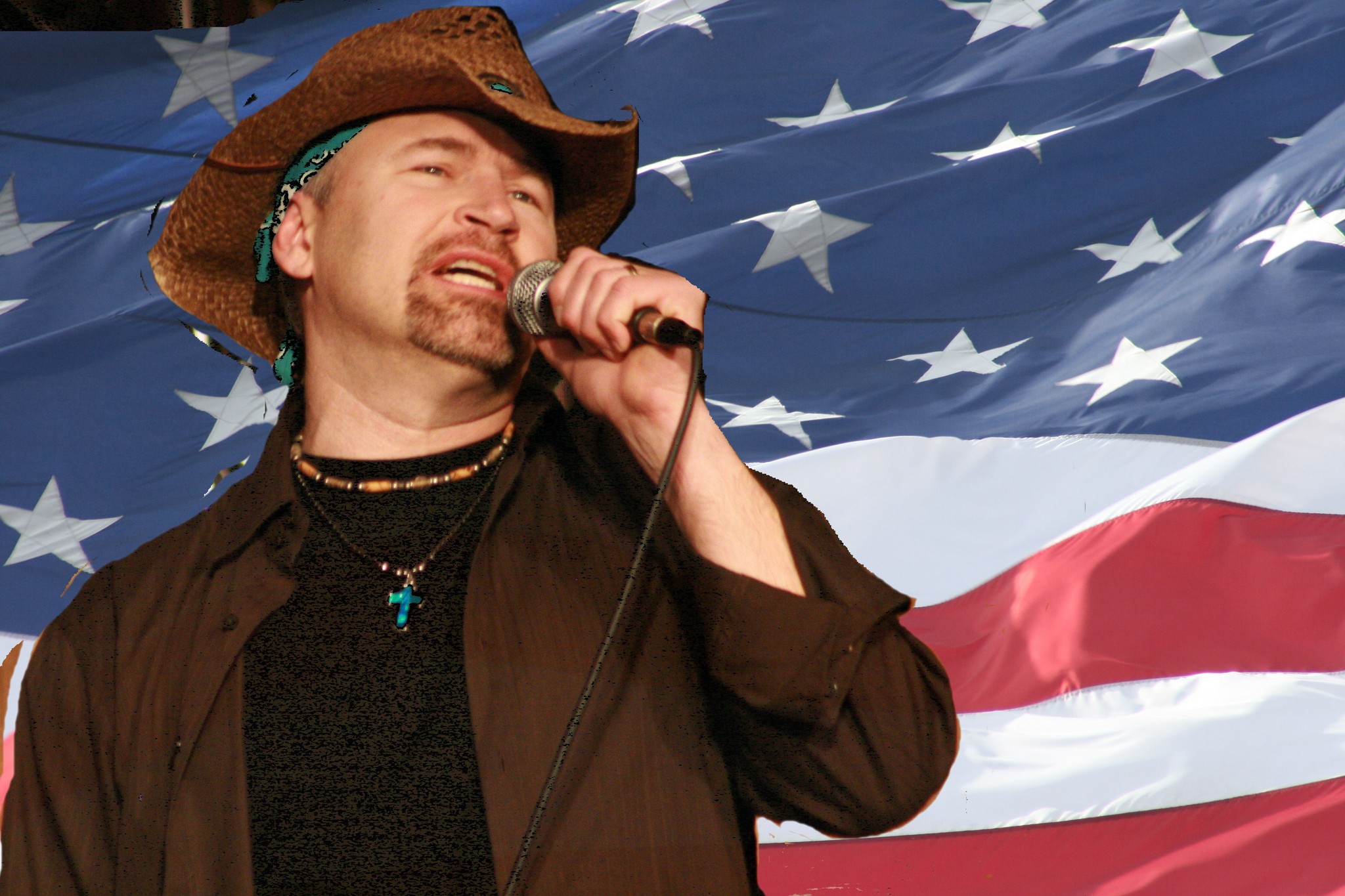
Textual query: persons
0,4,962,895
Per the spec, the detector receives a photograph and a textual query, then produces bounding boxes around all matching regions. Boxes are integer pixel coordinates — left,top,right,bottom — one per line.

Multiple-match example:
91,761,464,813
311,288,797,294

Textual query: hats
147,5,641,358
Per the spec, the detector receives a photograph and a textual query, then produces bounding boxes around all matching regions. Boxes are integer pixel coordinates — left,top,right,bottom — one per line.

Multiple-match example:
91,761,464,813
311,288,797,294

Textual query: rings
627,265,639,276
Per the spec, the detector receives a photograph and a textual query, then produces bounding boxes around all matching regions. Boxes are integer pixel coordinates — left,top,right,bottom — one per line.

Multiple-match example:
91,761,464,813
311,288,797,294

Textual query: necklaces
289,420,516,629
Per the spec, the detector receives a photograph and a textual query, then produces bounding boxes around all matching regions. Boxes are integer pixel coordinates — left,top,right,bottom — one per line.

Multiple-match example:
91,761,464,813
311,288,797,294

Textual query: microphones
506,260,701,349
